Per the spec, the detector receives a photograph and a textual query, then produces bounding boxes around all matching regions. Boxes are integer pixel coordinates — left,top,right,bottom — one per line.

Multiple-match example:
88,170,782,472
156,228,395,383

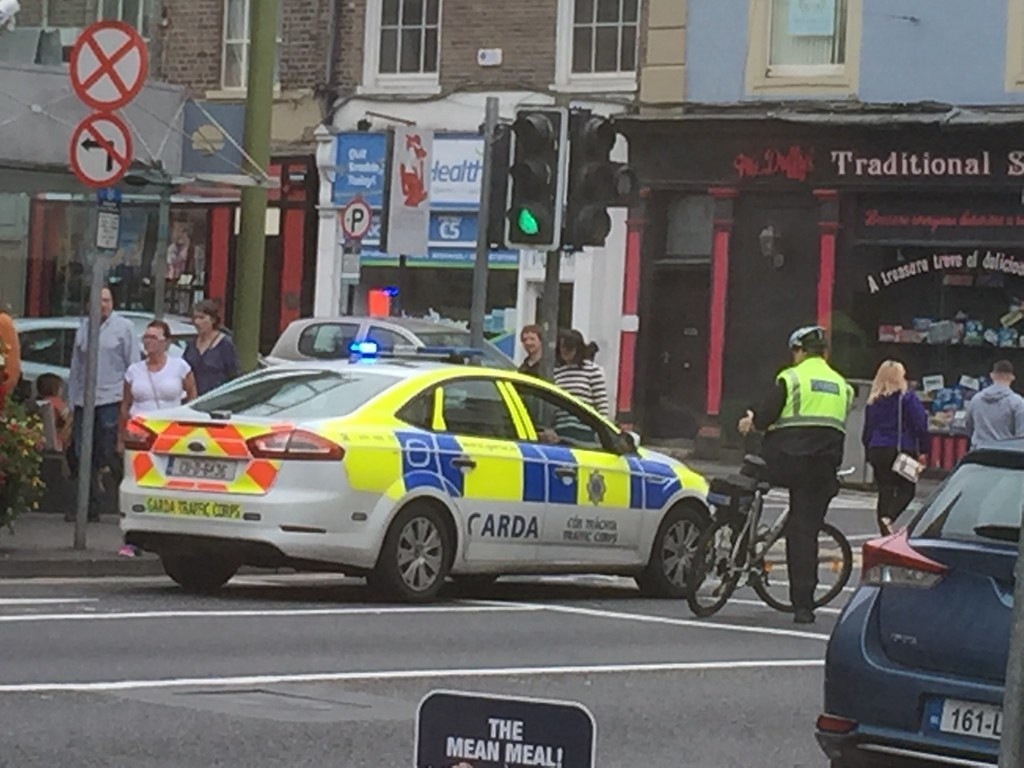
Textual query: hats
993,359,1014,372
787,326,827,353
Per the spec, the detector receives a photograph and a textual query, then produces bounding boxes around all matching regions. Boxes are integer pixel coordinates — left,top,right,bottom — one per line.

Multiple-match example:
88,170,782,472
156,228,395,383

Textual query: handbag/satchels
891,452,925,484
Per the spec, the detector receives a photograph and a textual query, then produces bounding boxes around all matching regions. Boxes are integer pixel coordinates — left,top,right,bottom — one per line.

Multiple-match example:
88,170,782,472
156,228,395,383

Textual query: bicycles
684,426,853,620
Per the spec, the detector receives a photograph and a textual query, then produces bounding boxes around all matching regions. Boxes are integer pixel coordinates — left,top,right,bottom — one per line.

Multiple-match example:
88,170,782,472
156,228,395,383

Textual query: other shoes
65,510,99,522
793,608,815,624
118,543,140,557
878,516,892,536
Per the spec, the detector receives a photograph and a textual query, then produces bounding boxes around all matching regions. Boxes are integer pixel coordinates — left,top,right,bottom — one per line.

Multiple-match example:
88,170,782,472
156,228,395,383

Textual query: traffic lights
563,111,616,248
505,102,568,253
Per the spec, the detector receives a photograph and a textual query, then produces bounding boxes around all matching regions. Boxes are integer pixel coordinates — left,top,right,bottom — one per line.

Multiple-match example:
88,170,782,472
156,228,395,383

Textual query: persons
552,329,608,441
0,310,20,525
64,281,141,522
25,372,67,451
184,300,243,395
115,320,197,557
737,323,855,624
965,360,1024,452
512,323,558,431
861,360,932,536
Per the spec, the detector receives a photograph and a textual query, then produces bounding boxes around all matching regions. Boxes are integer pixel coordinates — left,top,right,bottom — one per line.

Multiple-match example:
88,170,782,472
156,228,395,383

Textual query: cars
813,437,1024,767
14,311,236,379
265,315,520,372
119,362,717,604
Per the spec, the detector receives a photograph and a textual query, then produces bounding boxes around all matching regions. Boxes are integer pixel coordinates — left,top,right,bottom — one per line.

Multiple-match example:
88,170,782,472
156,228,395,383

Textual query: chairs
447,399,511,436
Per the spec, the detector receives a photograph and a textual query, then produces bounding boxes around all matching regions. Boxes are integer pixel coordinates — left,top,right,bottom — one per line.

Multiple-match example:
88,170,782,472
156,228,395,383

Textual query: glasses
141,335,166,343
